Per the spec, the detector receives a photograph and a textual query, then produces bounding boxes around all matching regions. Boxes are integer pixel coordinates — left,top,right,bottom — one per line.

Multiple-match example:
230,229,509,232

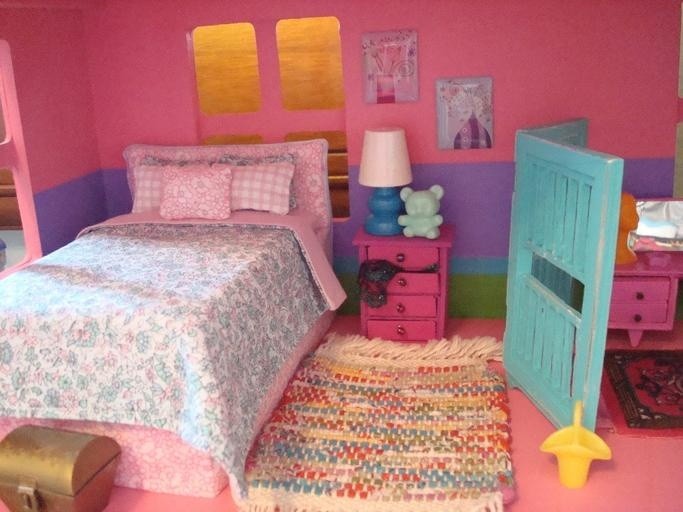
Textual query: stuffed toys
394,176,446,240
